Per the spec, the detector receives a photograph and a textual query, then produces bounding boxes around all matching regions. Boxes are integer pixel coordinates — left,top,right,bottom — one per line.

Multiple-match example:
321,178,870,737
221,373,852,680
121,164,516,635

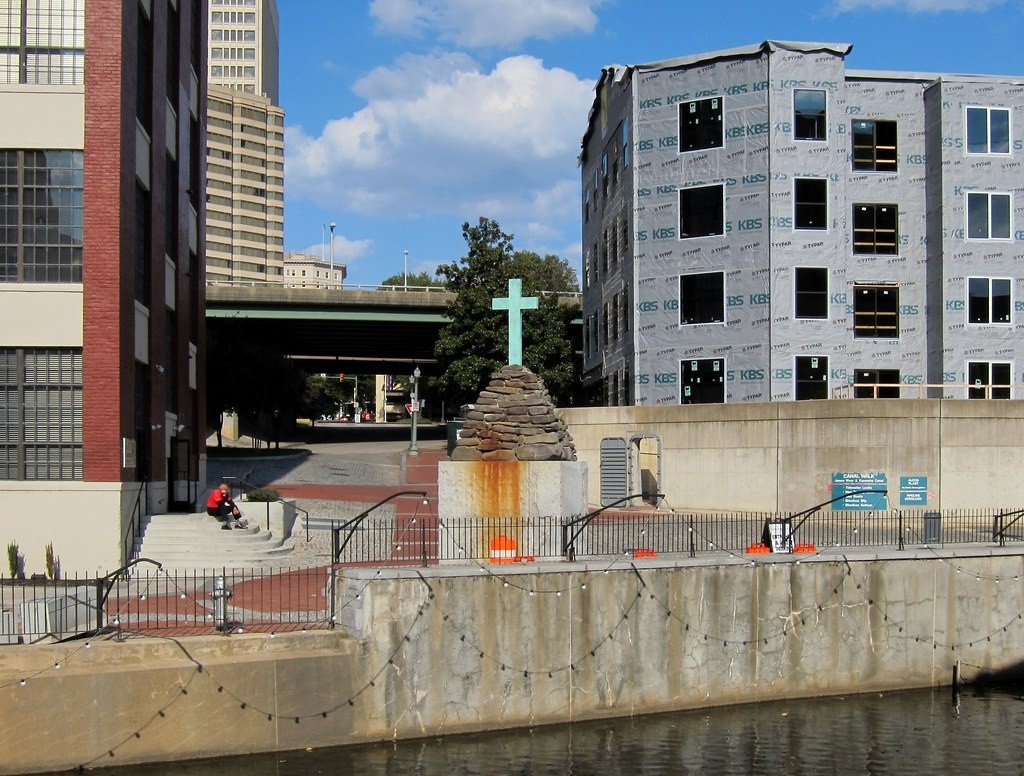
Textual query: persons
206,482,249,530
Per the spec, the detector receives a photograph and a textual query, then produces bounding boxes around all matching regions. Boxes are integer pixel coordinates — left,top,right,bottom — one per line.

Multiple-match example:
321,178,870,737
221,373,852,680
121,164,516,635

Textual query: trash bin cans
447,420,465,457
924,512,941,544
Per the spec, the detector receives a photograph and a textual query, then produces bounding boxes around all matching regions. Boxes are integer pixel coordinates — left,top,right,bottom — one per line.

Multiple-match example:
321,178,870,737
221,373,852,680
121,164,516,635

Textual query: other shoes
222,525,232,530
235,524,248,529
